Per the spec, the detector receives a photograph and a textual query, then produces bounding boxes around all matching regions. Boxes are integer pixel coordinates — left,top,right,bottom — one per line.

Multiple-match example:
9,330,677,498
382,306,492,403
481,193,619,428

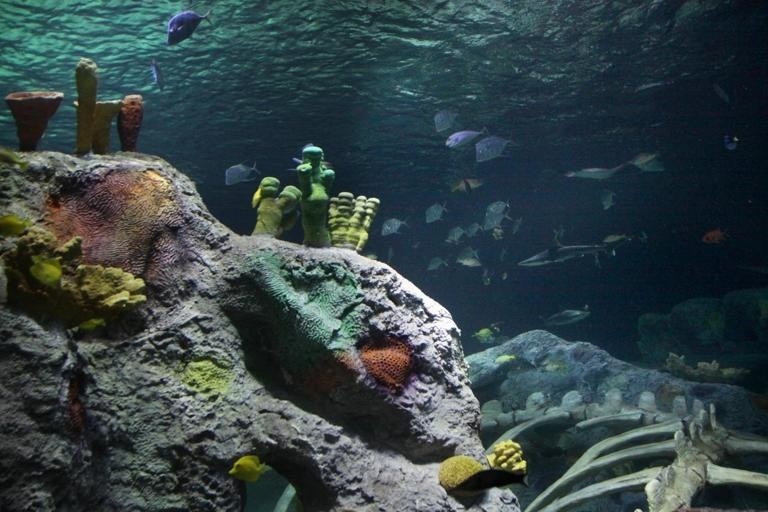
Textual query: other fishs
424,108,666,287
